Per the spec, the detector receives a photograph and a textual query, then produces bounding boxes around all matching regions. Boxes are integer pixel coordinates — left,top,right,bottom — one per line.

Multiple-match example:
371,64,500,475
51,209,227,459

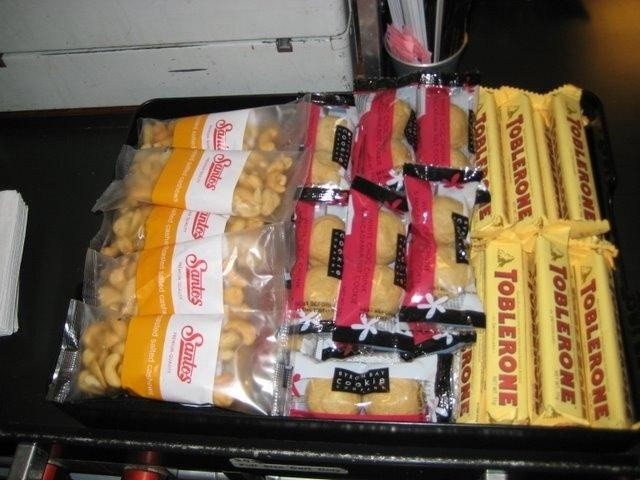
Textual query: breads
296,98,474,422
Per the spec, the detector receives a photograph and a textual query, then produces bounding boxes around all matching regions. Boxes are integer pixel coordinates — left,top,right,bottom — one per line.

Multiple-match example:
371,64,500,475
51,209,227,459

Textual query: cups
382,20,469,84
